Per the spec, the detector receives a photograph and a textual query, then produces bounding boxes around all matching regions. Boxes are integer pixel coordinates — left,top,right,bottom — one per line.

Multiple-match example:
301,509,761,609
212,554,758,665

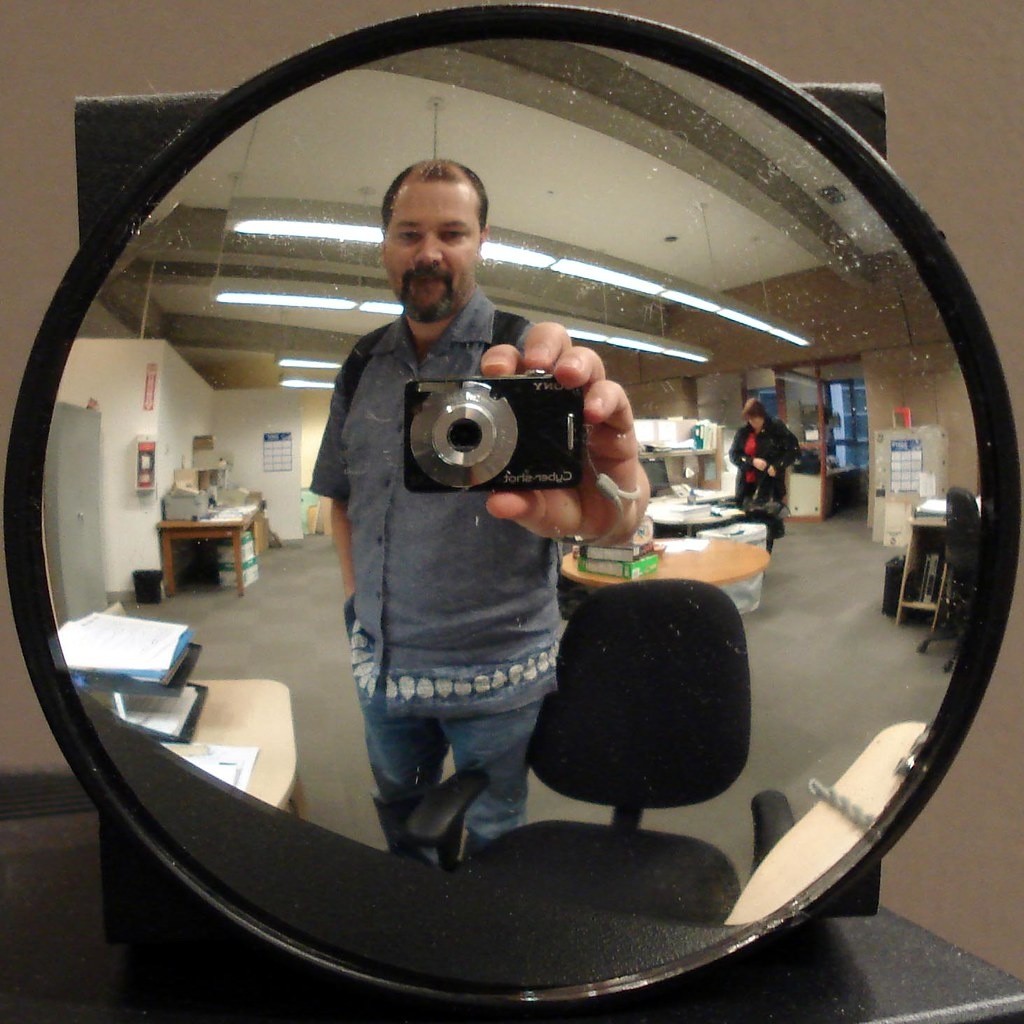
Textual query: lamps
210,198,814,391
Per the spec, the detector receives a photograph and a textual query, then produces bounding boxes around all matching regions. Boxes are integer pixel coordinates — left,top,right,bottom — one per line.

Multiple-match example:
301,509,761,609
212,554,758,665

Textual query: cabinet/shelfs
633,416,726,507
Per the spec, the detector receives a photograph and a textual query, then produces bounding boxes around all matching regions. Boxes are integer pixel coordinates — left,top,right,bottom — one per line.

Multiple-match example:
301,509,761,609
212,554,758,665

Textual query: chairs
914,486,984,672
410,578,796,927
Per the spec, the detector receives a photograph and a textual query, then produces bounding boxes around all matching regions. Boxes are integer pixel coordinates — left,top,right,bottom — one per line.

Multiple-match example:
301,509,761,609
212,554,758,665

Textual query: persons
309,159,651,874
728,398,800,580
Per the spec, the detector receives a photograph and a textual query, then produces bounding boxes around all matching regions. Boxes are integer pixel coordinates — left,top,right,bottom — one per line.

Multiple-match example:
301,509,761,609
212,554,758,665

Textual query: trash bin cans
133,569,164,606
881,553,905,619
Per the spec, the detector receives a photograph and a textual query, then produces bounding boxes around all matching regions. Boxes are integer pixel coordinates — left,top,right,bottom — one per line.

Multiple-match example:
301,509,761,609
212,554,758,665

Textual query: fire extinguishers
138,441,154,489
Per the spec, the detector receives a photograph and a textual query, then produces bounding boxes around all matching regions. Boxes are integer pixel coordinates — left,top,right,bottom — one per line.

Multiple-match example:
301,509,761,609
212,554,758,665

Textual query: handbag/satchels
745,470,791,523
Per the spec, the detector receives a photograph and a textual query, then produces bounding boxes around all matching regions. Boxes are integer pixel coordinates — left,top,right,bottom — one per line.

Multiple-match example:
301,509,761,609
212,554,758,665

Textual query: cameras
403,369,585,494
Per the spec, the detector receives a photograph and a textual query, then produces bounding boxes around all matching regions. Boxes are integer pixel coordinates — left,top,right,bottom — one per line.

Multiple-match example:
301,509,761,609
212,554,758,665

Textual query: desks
560,537,770,589
0,770,1024,1024
156,502,266,596
894,497,981,626
646,500,744,540
157,679,307,819
